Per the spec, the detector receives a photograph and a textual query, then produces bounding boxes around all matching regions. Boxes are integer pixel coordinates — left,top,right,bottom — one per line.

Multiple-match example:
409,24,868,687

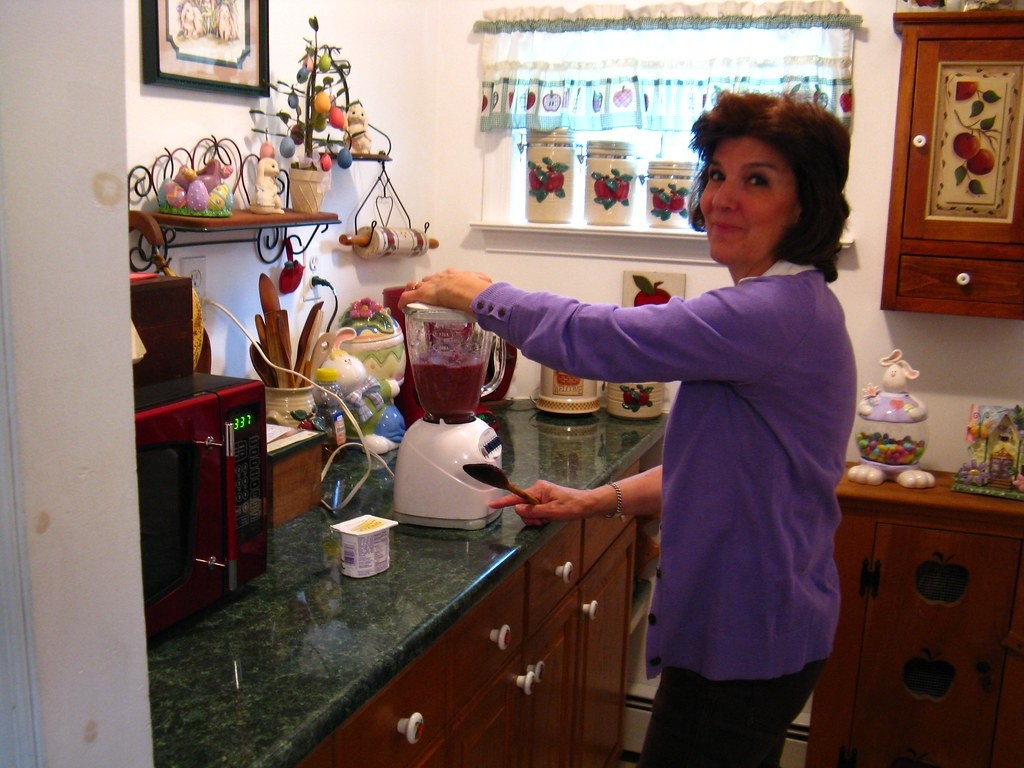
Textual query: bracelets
601,481,622,520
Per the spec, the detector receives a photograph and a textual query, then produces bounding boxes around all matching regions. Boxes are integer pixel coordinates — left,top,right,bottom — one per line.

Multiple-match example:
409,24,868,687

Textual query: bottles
524,125,573,222
312,368,346,463
645,159,700,230
585,139,635,226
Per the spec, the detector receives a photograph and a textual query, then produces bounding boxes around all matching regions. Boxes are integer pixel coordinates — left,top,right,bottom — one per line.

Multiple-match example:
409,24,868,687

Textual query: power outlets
300,245,325,300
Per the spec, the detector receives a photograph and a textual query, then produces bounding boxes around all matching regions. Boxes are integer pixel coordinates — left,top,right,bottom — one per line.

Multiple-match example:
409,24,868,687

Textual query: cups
535,363,600,414
263,385,315,429
607,381,664,419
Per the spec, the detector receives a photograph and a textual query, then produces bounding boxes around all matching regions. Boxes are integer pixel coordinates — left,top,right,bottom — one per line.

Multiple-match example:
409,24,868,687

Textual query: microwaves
133,371,273,641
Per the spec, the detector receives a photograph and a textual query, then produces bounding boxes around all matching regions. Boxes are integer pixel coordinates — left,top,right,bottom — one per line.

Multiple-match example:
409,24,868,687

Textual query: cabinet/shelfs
524,458,642,768
805,463,1024,768
880,11,1024,321
335,565,527,768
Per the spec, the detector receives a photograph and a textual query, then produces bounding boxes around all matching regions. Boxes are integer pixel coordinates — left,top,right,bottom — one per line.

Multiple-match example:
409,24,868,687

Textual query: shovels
463,462,541,506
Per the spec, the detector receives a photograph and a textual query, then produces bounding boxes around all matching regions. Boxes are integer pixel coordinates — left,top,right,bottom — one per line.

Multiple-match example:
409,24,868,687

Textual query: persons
399,92,861,767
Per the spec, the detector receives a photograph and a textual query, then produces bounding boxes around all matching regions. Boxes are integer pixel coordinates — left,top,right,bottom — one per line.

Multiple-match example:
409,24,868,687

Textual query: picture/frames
140,0,270,97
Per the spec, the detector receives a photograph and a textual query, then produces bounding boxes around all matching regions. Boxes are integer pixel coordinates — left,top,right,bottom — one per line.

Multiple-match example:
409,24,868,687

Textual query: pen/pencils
330,477,345,519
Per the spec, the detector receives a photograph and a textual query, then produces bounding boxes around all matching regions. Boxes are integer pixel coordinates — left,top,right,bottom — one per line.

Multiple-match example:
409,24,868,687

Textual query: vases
284,164,330,213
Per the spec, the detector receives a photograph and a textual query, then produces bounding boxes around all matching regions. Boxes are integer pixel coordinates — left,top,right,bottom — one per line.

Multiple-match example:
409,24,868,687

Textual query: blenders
392,300,519,532
